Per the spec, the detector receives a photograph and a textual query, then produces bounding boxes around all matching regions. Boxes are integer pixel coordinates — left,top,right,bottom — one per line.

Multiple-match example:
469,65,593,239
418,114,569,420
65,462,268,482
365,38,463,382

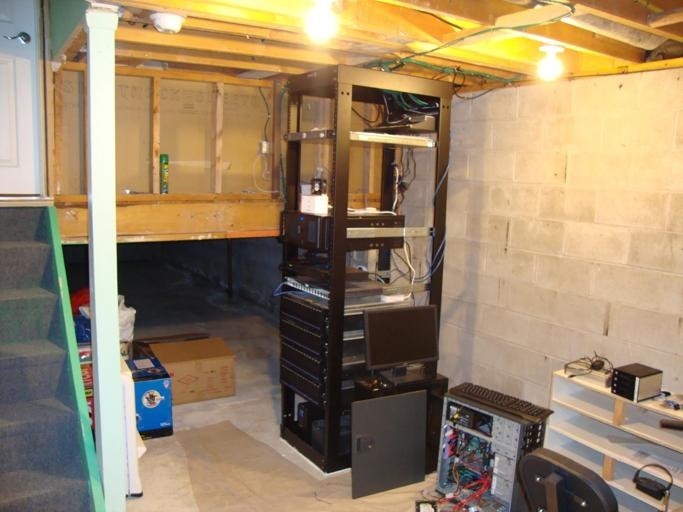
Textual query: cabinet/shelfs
279,65,453,474
543,367,683,512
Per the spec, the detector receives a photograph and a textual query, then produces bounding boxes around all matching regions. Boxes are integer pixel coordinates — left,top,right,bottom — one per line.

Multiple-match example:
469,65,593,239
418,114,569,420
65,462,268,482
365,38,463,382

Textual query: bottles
311,178,321,196
160,155,169,195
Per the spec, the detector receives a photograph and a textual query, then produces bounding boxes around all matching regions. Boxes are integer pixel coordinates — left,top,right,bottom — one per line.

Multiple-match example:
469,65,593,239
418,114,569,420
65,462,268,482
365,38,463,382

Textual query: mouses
590,360,604,370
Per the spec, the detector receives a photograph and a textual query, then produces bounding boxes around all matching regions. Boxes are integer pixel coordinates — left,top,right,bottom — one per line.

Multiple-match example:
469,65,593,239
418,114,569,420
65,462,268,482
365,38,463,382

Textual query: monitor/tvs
363,304,439,386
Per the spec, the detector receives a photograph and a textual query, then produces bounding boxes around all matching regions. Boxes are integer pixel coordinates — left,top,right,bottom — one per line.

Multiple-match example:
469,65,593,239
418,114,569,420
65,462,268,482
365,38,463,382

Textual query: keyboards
449,381,554,423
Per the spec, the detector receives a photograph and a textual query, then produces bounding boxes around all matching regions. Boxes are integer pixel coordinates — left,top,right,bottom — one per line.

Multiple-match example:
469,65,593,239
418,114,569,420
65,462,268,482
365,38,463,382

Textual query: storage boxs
126,356,173,440
133,332,235,406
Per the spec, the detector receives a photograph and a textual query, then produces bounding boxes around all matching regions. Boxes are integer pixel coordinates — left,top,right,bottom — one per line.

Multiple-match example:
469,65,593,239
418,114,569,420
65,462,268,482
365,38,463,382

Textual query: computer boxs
435,391,546,512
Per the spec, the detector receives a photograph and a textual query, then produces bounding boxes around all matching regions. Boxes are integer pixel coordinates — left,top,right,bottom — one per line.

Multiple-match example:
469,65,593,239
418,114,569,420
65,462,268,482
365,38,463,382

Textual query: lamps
305,0,338,41
537,46,565,82
